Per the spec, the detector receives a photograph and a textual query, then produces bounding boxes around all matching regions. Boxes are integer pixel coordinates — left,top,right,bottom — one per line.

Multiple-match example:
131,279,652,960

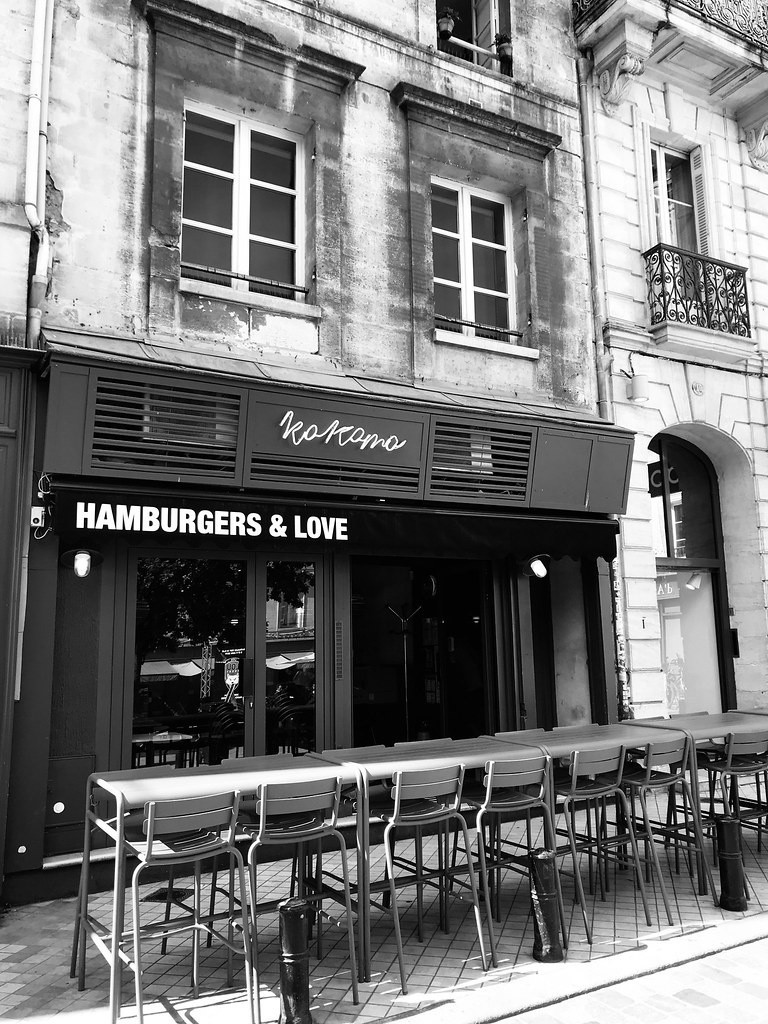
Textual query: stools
67,712,768,1024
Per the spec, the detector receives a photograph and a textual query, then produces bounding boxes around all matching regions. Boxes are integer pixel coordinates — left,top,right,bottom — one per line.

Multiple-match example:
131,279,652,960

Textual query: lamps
517,543,552,578
58,529,108,579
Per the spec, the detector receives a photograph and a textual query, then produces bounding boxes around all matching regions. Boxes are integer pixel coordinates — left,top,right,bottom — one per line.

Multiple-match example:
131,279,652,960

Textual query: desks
322,735,557,983
494,722,709,896
131,725,210,769
69,750,365,991
622,708,768,886
728,709,768,715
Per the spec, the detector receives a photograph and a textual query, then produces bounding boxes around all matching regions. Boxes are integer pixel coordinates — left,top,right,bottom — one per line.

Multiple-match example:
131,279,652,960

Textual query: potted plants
494,33,512,57
437,6,463,40
